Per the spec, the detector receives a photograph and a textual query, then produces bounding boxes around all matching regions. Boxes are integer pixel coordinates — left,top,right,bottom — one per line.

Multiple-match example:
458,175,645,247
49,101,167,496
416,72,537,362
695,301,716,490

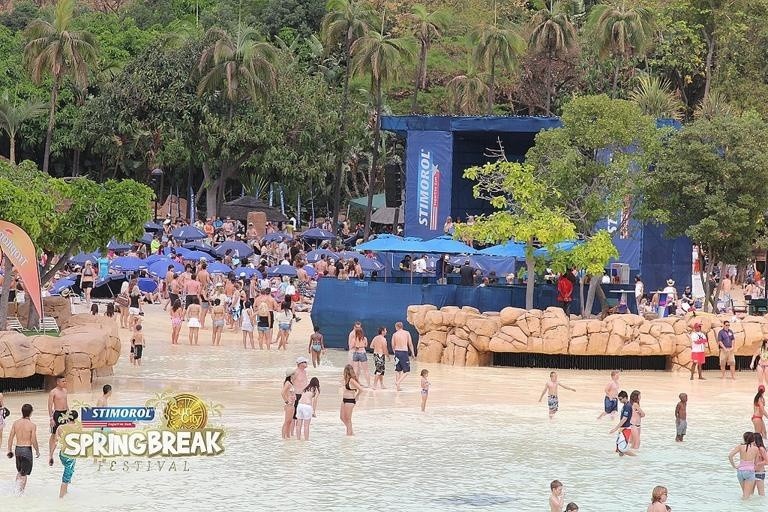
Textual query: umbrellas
50,191,610,303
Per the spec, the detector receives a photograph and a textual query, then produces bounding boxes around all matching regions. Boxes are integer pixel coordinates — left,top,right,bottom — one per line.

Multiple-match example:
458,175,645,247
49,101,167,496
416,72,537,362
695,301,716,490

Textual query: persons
0,207,768,512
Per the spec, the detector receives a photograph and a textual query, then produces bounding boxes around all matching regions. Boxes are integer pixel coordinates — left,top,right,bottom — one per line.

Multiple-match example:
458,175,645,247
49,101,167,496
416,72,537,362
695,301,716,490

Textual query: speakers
384,163,402,207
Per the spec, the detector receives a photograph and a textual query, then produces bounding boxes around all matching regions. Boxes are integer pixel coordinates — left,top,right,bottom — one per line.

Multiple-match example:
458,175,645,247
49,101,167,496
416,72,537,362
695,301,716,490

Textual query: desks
650,291,674,306
609,290,636,306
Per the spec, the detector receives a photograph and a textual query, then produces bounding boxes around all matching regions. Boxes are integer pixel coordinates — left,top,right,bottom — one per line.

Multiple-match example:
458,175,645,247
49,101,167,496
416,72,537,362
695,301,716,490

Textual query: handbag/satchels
115,295,129,307
286,286,295,296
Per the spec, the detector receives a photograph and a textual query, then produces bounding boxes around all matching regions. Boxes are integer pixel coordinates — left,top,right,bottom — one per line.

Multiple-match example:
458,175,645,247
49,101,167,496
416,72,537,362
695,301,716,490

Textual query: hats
62,288,71,297
44,281,52,289
465,260,469,263
72,264,81,271
296,357,307,364
240,272,245,276
694,323,702,327
667,279,675,286
286,369,294,377
759,385,765,392
200,257,206,263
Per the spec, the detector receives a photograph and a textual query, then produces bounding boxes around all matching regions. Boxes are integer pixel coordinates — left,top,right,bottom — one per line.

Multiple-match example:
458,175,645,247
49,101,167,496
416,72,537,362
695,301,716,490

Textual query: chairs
31,317,61,333
730,298,748,315
6,316,25,333
